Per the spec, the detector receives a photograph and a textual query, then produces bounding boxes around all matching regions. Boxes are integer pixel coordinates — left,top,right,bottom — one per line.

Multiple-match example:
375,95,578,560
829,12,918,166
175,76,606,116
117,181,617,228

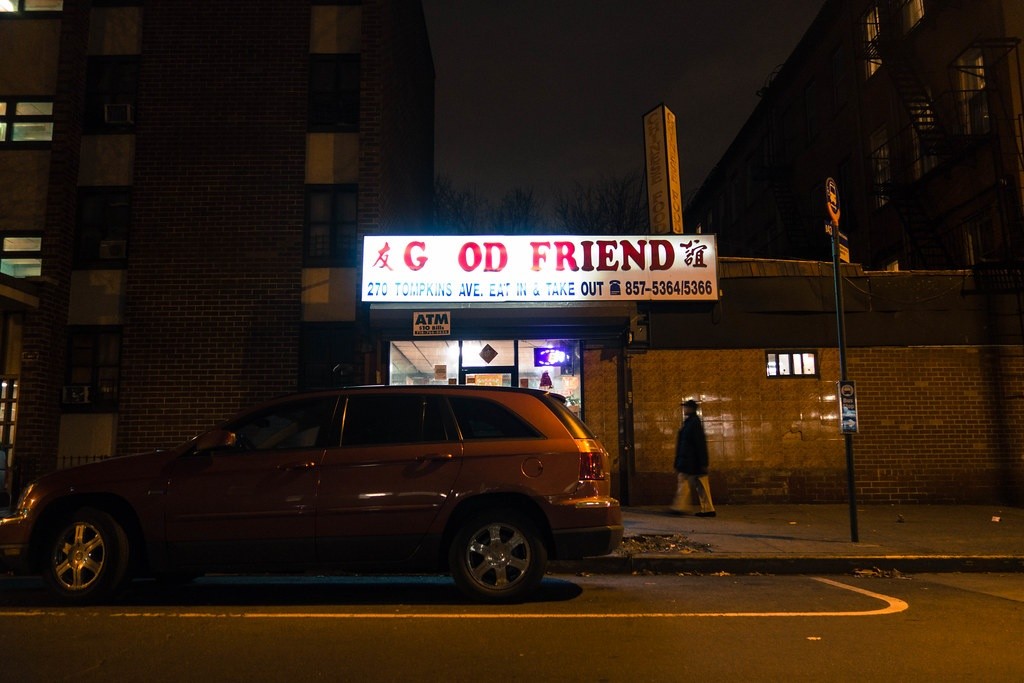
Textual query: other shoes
694,511,716,517
665,511,686,516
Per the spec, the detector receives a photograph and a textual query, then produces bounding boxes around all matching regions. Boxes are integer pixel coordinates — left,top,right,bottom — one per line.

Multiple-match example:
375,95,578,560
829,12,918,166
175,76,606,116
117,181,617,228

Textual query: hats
681,400,698,409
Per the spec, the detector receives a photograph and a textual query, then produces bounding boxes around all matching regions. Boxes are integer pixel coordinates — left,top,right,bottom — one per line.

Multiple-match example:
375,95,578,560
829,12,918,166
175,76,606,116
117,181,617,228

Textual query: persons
667,400,716,518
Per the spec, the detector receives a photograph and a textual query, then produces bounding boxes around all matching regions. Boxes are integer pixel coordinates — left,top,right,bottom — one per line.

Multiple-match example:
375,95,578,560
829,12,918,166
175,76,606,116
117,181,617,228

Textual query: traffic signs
837,380,859,434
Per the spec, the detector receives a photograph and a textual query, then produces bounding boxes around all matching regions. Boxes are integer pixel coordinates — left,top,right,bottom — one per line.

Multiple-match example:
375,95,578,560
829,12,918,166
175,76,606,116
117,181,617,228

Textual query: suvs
0,385,625,609
